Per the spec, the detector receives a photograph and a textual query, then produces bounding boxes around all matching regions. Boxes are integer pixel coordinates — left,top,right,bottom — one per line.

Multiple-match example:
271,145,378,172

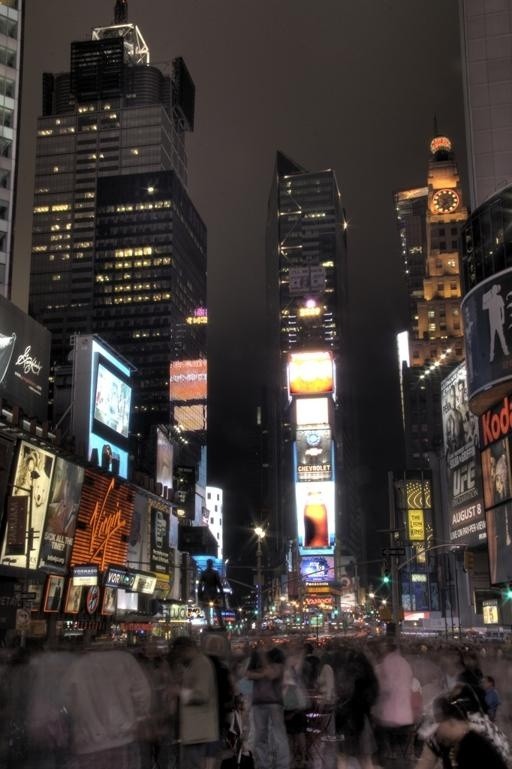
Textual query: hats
417,697,462,739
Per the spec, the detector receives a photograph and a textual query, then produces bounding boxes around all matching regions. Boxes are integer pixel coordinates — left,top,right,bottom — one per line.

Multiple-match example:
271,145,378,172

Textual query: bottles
305,489,327,551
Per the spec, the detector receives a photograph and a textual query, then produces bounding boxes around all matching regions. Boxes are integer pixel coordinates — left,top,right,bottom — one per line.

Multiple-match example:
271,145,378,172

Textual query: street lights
254,526,265,635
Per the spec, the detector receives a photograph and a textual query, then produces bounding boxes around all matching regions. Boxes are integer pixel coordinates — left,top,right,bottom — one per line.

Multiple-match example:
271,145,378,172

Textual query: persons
482,284,510,363
0,624,512,769
494,454,507,500
198,559,224,627
443,379,477,450
